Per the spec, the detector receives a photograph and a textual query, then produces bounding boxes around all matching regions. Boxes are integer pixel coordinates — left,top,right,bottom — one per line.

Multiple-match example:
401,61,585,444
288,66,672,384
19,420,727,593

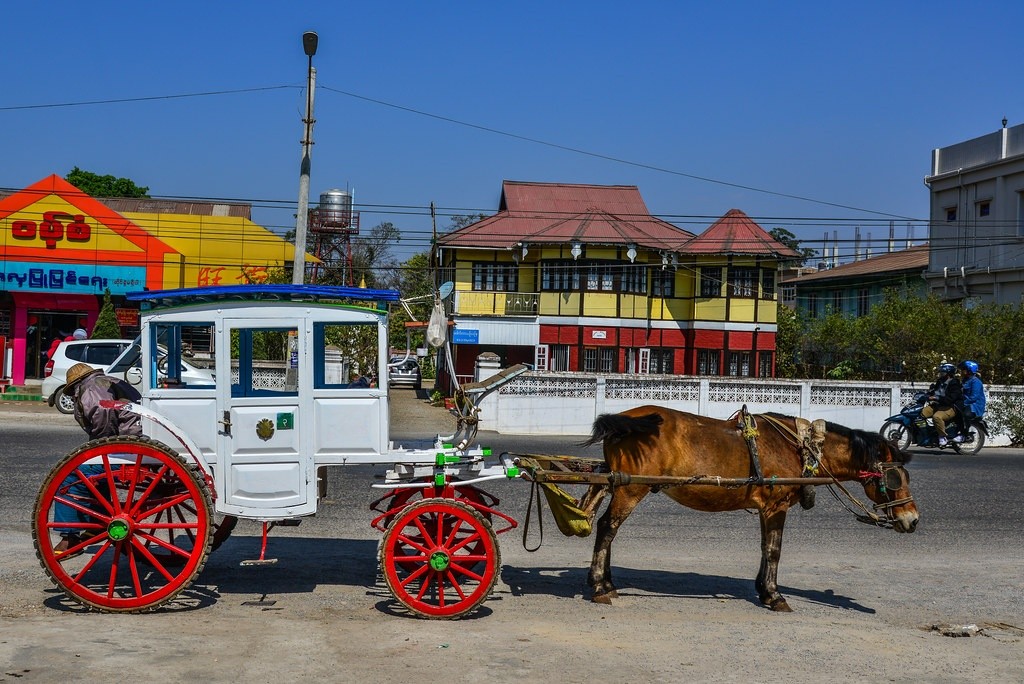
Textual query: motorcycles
878,382,991,456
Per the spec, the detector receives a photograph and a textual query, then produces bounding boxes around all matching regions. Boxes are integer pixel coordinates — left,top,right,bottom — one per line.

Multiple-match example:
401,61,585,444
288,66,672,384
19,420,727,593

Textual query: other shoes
53,536,84,561
81,531,106,544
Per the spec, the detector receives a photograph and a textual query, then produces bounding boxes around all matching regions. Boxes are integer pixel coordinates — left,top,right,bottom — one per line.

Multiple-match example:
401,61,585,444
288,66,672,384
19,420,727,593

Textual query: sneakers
939,437,948,446
952,435,965,443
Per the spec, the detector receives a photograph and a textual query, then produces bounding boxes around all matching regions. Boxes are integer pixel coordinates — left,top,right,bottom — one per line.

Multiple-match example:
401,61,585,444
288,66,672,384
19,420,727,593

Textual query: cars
388,359,421,390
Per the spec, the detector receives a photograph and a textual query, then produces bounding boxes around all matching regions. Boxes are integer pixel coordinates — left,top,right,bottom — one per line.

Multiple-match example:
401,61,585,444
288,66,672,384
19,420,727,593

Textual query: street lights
291,32,319,285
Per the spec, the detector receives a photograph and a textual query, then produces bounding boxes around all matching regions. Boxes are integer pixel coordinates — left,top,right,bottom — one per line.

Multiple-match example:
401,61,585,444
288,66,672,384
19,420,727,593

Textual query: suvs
41,339,216,414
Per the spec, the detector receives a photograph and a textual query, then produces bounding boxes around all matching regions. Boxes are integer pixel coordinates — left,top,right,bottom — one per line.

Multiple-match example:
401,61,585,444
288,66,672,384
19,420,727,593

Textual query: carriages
29,284,923,622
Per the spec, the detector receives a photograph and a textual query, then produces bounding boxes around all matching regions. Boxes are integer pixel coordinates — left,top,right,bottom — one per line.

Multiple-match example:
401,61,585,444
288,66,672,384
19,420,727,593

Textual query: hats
62,363,104,396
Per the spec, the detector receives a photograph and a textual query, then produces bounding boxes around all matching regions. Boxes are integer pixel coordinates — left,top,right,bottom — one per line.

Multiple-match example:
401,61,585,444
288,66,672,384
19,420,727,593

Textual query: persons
53,363,143,561
952,360,985,442
351,373,367,385
920,364,964,445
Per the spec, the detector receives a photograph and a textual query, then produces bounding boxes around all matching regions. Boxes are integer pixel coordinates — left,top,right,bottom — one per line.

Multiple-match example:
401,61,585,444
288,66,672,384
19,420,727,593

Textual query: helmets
959,361,978,375
938,364,957,377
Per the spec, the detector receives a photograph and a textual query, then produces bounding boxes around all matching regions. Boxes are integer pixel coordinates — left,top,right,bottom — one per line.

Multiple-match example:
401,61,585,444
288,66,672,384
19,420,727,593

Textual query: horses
572,405,919,613
